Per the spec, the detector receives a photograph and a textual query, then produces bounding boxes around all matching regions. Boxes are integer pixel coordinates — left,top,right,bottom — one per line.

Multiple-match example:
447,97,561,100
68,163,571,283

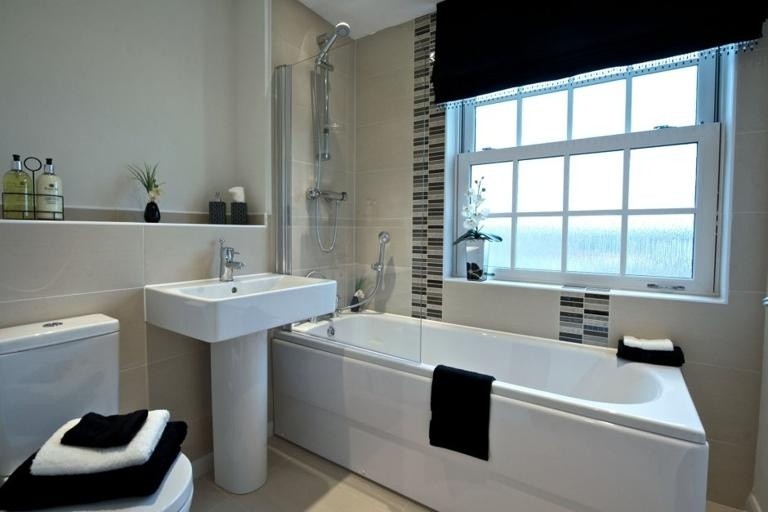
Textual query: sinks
143,272,337,344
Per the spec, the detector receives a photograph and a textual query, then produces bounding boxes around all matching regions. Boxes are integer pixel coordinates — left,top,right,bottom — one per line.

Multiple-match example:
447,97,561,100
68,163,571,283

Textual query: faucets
219,239,245,281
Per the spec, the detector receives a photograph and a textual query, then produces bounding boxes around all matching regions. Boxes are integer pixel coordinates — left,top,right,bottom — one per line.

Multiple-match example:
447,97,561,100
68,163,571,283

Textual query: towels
0,410,187,512
616,338,686,366
428,366,493,461
227,186,245,203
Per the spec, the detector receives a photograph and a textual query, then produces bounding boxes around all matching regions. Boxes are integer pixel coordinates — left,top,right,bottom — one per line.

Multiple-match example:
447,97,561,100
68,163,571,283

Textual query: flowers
350,279,364,298
451,175,503,246
126,161,163,201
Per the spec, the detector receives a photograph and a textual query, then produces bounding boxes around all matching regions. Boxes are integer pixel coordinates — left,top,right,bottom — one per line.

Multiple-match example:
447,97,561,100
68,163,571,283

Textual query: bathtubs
0,313,195,512
293,307,707,447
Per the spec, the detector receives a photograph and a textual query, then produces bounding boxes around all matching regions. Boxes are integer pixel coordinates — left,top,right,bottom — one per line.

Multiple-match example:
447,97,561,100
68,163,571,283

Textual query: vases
144,201,161,223
350,296,360,312
464,239,485,280
